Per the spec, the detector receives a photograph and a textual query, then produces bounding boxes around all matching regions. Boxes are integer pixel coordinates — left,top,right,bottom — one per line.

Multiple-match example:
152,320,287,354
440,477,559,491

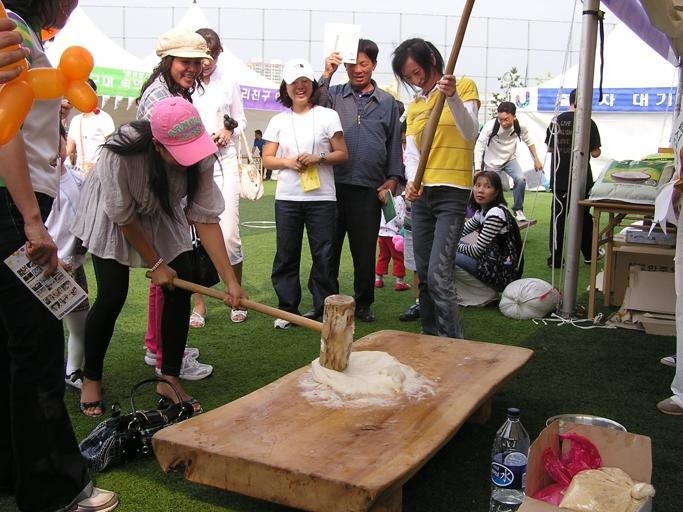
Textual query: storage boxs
608,234,677,308
523,419,654,512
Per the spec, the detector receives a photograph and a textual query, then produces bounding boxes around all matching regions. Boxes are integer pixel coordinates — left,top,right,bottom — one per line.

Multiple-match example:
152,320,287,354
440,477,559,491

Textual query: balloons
1,1,100,145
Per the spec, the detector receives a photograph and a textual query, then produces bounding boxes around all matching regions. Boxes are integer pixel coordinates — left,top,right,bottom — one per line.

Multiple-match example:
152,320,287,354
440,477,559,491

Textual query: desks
579,195,654,321
151,331,535,510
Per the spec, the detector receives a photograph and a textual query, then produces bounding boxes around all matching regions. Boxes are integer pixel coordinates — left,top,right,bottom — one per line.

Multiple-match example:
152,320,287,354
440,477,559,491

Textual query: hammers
145,270,357,371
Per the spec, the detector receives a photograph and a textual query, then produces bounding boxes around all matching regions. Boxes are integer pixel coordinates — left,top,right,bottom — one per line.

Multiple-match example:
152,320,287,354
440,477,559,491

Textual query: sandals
189,305,207,327
231,309,247,323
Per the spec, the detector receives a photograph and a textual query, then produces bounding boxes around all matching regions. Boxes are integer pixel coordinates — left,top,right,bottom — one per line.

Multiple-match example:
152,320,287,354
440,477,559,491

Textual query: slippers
79,397,104,418
154,391,203,414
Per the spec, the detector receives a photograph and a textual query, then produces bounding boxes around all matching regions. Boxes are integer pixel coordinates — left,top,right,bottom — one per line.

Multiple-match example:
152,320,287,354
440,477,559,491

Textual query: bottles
485,407,531,512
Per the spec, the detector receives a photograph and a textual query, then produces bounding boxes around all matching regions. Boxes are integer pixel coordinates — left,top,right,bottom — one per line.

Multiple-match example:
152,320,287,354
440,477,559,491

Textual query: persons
134,28,213,382
392,38,481,340
398,122,420,322
1,1,119,512
189,28,249,328
454,171,523,294
375,195,412,290
474,102,543,221
0,19,31,84
544,88,605,268
261,60,350,329
66,78,116,170
68,96,249,415
303,39,404,322
44,123,89,388
252,130,272,179
656,49,683,415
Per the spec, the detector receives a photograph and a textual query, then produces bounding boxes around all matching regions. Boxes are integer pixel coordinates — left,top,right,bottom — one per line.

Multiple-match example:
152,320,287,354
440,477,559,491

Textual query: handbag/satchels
161,242,219,300
237,163,265,201
78,379,194,474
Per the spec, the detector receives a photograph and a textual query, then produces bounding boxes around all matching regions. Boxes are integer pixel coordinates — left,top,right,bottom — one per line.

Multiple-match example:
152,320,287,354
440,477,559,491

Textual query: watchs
320,152,326,160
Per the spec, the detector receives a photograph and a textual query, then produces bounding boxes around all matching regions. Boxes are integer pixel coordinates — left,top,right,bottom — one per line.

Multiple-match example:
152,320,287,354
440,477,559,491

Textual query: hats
150,97,218,166
156,29,214,62
283,59,315,84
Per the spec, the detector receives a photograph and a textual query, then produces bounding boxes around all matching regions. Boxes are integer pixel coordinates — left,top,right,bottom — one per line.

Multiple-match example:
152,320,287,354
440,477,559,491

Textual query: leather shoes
302,309,321,320
356,308,375,322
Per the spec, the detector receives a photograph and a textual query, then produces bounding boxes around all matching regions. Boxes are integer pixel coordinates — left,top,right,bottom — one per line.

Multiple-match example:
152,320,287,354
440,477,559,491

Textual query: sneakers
145,346,199,365
154,355,213,380
375,280,383,287
395,283,411,290
657,398,682,415
67,487,119,511
514,210,527,222
65,369,83,388
584,249,605,263
660,355,677,367
399,304,420,321
274,318,292,329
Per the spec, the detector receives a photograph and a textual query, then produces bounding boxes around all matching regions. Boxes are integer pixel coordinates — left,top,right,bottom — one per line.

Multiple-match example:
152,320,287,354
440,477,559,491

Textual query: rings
220,137,225,141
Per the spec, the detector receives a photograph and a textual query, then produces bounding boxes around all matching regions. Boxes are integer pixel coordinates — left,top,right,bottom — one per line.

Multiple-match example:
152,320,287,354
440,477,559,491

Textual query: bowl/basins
610,169,651,184
545,411,627,439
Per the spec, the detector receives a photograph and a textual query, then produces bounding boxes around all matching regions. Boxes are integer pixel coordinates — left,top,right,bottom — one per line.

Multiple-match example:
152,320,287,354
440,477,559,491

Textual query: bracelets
151,257,164,272
